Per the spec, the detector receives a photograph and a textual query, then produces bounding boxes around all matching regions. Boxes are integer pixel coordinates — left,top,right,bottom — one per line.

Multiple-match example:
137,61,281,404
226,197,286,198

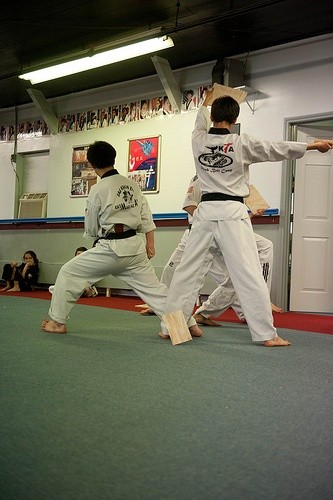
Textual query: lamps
16,26,180,86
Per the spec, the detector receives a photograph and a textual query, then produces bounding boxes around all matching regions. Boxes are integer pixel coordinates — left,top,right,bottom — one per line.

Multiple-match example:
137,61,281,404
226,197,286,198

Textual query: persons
42,140,203,338
0,251,40,292
49,247,99,298
139,87,333,347
0,87,207,143
